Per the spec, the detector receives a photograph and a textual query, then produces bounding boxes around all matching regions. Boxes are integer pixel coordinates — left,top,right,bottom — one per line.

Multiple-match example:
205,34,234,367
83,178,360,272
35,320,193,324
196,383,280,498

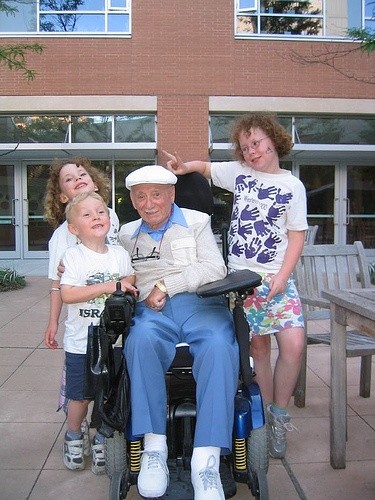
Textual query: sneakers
137,442,169,498
266,405,299,458
80,419,91,457
191,455,225,500
63,431,85,470
91,434,107,475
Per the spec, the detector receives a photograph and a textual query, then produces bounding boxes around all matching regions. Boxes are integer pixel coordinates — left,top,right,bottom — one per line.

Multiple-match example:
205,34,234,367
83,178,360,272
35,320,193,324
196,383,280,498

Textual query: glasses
132,247,161,263
241,136,270,154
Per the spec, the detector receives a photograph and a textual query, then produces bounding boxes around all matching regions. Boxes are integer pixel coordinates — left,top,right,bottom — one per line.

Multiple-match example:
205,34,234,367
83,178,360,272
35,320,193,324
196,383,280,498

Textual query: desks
320,289,375,470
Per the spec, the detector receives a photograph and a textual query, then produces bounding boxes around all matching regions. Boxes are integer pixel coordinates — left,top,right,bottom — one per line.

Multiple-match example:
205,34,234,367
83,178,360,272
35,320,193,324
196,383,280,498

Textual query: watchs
153,279,169,294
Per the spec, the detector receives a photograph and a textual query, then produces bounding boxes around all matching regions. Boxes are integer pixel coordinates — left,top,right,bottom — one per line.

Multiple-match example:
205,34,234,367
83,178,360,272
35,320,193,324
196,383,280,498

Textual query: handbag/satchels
91,349,130,434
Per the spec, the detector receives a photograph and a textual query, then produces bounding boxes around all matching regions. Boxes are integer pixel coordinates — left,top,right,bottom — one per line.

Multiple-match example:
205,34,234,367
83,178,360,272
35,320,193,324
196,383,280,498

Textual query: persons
43,158,138,475
55,165,240,500
160,115,308,460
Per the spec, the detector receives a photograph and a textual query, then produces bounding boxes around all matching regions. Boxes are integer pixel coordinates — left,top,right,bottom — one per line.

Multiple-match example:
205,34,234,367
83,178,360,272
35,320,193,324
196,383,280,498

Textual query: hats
125,165,177,190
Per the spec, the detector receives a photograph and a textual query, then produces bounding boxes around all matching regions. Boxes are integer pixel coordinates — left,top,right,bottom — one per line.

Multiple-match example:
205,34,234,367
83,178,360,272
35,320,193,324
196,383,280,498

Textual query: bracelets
49,288,60,293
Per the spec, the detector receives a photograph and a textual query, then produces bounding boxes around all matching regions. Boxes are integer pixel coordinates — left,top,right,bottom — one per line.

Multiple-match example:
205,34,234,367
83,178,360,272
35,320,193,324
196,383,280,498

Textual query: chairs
295,241,375,406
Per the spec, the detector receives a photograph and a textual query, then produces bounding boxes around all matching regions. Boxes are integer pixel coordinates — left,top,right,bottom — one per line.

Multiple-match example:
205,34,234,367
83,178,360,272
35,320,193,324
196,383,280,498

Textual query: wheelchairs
86,168,272,500
